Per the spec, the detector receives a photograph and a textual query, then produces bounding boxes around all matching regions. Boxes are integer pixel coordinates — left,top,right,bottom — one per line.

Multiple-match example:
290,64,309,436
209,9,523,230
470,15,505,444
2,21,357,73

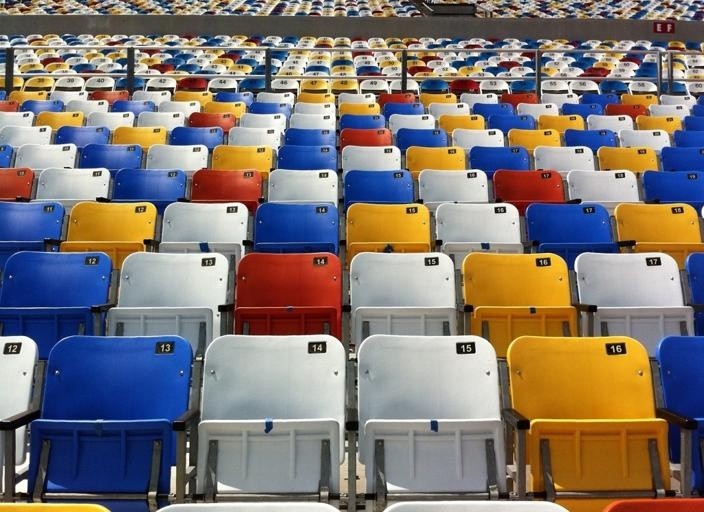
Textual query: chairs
0,0,704,20
1,34,703,512
